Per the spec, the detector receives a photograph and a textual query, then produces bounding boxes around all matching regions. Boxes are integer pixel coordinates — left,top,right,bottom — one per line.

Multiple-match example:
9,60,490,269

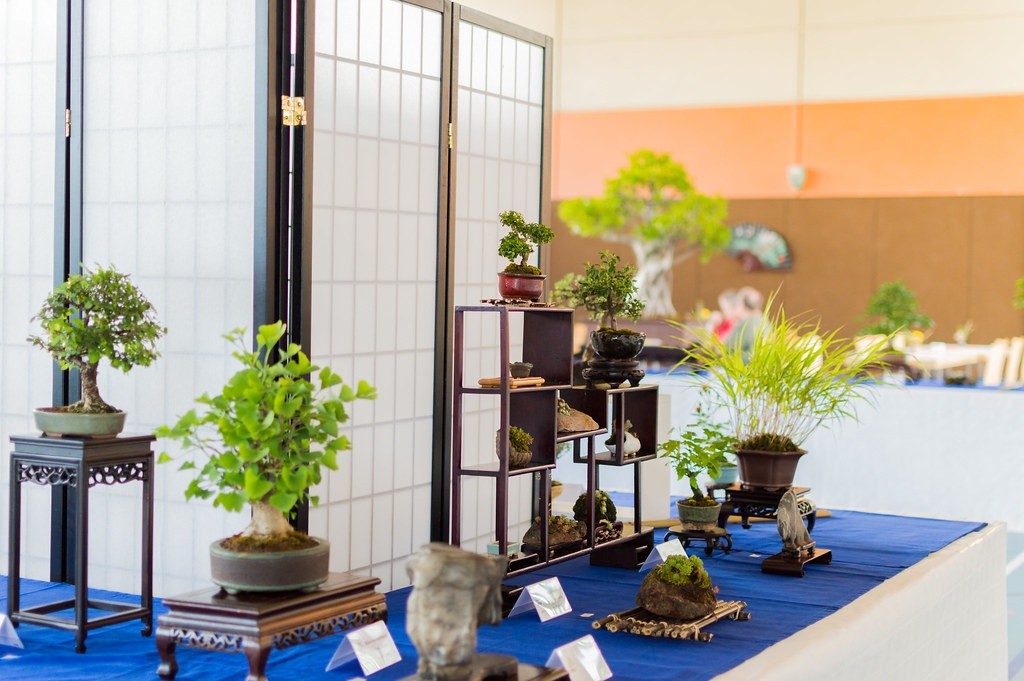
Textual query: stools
705,480,741,498
154,571,388,680
717,485,817,539
662,526,731,556
4,434,156,653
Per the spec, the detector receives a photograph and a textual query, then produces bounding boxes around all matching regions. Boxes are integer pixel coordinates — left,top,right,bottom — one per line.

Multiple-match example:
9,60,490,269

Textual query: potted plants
656,276,931,491
26,262,169,437
148,319,377,600
495,425,534,469
558,249,647,387
656,416,742,530
557,143,733,350
633,549,716,621
496,211,554,302
854,277,936,352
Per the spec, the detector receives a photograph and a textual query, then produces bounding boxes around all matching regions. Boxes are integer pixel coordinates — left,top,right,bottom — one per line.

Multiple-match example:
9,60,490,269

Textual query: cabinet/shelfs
451,306,660,616
